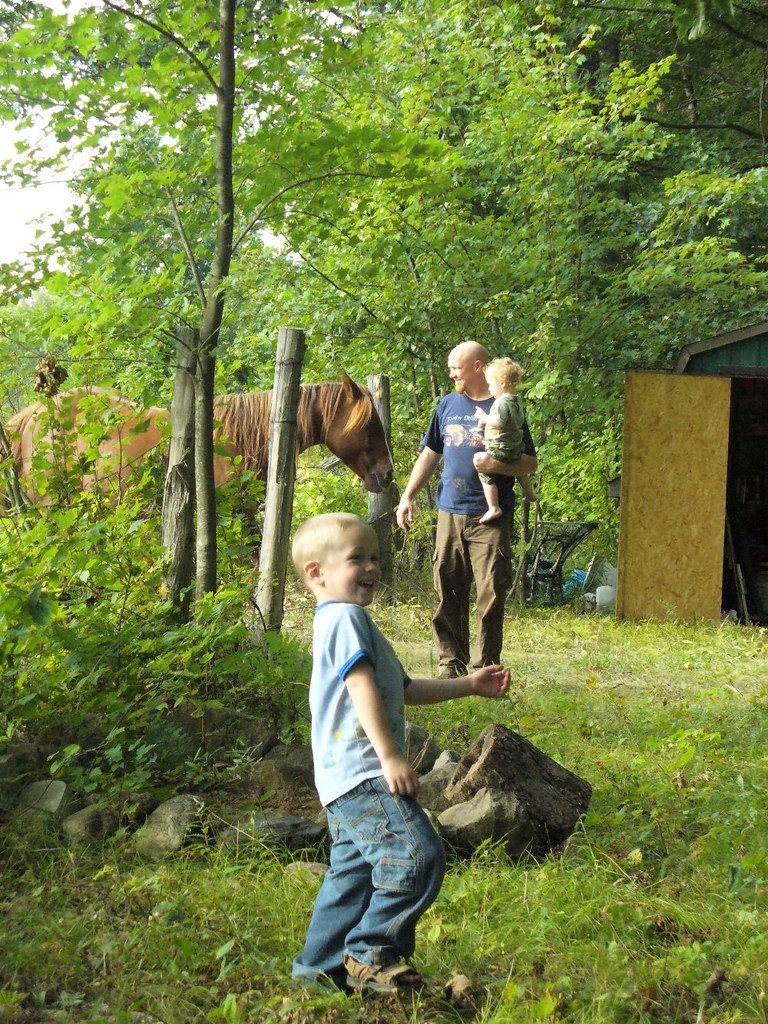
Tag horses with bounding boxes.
[2,370,393,566]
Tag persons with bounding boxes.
[292,512,511,993]
[478,356,537,524]
[397,341,537,679]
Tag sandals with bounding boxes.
[343,954,425,994]
